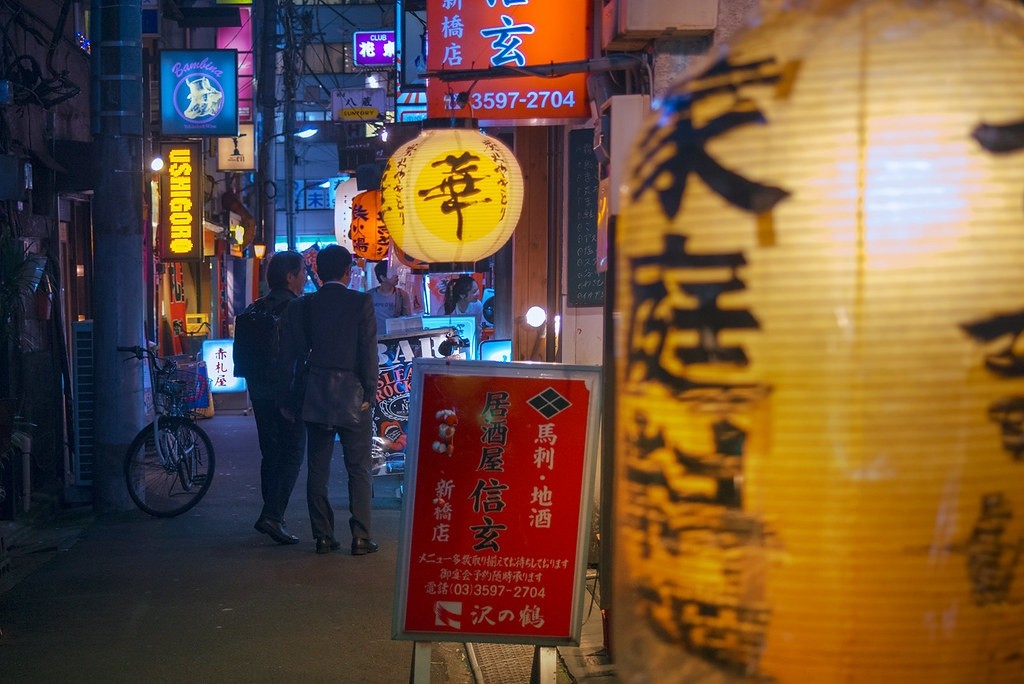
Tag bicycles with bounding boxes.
[172,319,211,355]
[114,344,217,518]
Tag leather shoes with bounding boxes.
[351,538,378,556]
[315,537,340,554]
[254,518,300,545]
[281,519,287,526]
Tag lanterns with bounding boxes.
[607,1,1023,684]
[333,126,524,264]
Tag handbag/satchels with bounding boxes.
[302,366,366,429]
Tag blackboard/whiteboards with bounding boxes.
[568,128,605,308]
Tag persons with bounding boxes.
[281,244,379,556]
[435,275,484,342]
[363,261,412,335]
[231,250,309,546]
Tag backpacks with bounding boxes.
[243,297,286,358]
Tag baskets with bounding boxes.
[154,366,212,415]
[179,331,208,357]
